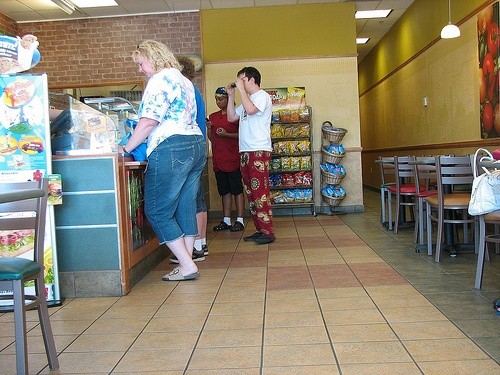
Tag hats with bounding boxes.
[214,86,229,97]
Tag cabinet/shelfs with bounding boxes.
[267,85,317,217]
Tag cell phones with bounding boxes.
[205,118,209,122]
[231,83,236,88]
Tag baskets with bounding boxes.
[320,163,347,185]
[320,143,347,165]
[320,185,347,207]
[321,120,349,144]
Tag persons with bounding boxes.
[143,57,209,262]
[225,65,276,245]
[202,87,246,232]
[117,39,206,280]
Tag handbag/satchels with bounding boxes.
[467,148,500,216]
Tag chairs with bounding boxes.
[413,153,459,245]
[426,155,481,262]
[377,156,415,223]
[0,178,59,375]
[471,155,500,289]
[387,155,426,234]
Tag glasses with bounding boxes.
[136,44,144,49]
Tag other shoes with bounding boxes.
[243,232,263,242]
[169,247,206,264]
[202,245,209,256]
[255,234,276,244]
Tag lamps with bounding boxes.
[441,0,460,38]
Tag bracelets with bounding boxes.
[122,147,130,154]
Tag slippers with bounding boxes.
[229,221,245,232]
[212,220,232,231]
[162,266,200,281]
